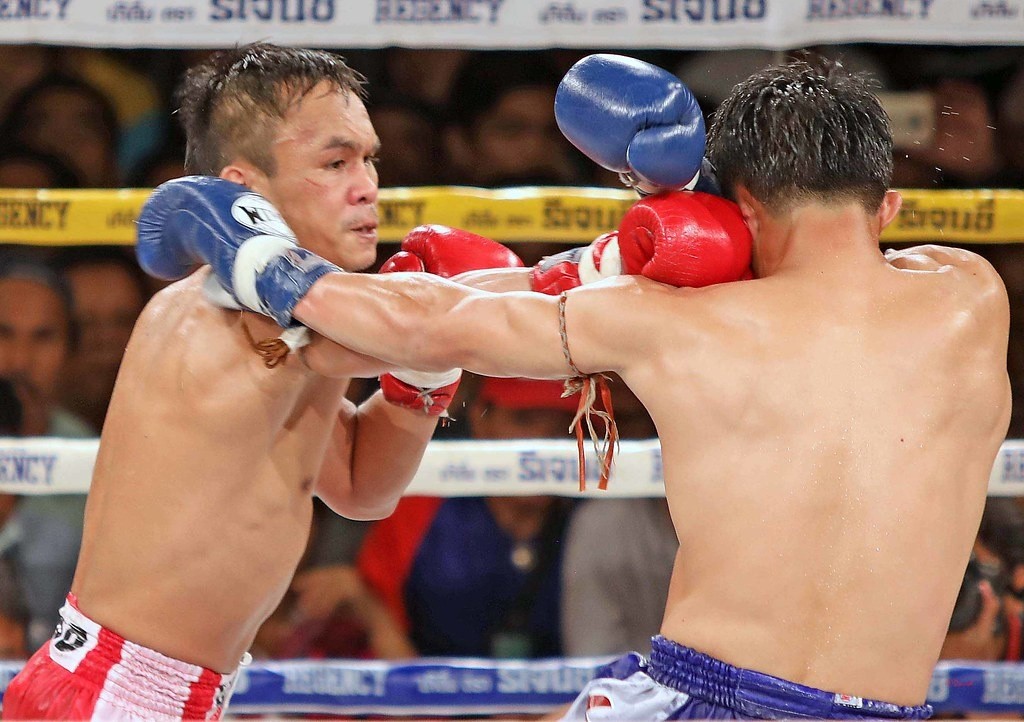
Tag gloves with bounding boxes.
[136,175,347,329]
[374,224,526,416]
[553,54,707,194]
[527,190,752,297]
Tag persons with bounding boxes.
[0,43,1024,661]
[135,55,1014,719]
[0,42,752,722]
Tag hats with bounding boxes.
[482,378,582,413]
